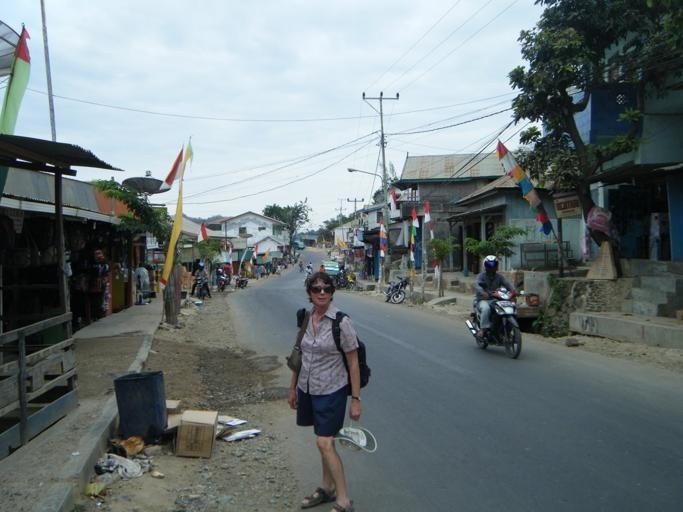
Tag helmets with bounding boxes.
[482,255,499,273]
[198,262,204,269]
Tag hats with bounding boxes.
[332,425,377,454]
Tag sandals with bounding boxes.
[333,500,354,512]
[301,487,336,509]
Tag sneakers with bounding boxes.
[477,330,484,337]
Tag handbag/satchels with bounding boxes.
[286,349,301,373]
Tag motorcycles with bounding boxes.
[235,275,248,290]
[465,287,529,359]
[194,275,208,299]
[382,272,411,304]
[216,274,230,292]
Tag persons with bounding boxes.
[471,254,518,338]
[282,270,363,511]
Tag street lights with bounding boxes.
[347,167,390,270]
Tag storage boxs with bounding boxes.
[175,410,218,458]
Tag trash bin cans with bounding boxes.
[113,370,168,445]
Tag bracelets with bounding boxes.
[350,395,361,401]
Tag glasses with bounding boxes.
[311,286,334,294]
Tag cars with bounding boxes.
[321,261,339,277]
[292,241,305,249]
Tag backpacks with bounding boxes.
[296,309,371,387]
